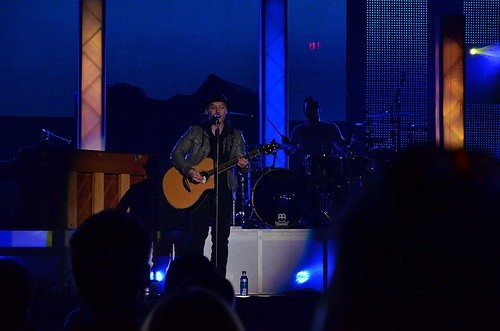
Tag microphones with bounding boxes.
[213,114,221,119]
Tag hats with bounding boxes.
[207,91,230,107]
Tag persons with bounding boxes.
[169,92,250,280]
[285,95,360,181]
[1,143,500,331]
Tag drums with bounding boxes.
[330,152,365,188]
[293,156,329,187]
[251,168,322,229]
[240,166,276,206]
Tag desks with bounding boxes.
[202,226,339,294]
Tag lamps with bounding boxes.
[39,128,72,146]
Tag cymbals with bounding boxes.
[246,143,302,150]
[367,111,415,116]
[366,136,388,143]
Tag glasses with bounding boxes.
[305,107,318,114]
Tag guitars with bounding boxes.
[162,142,280,211]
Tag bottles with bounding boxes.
[240,271,248,295]
